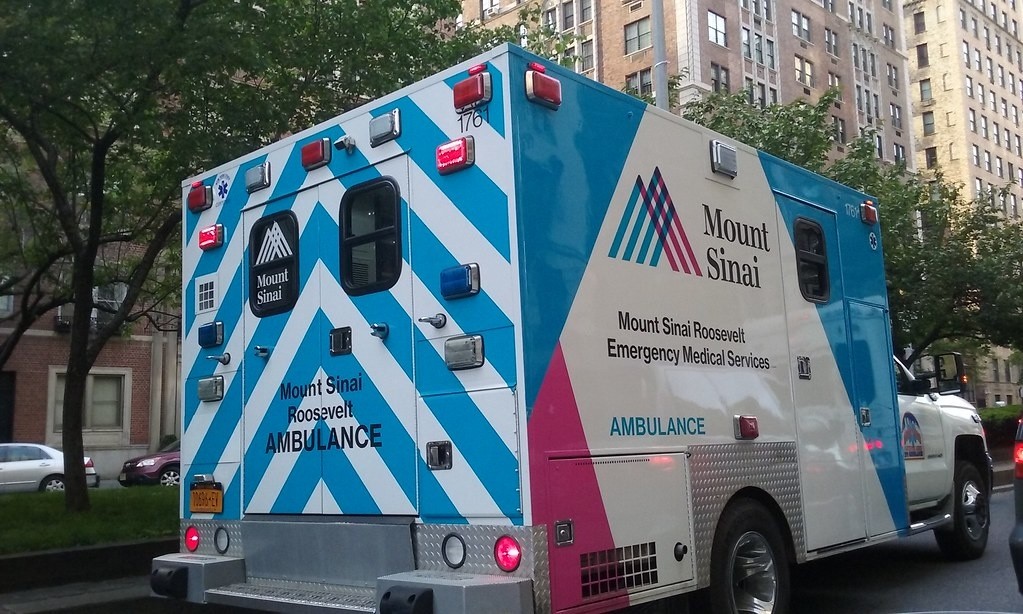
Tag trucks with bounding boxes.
[149,41,995,614]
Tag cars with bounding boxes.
[116,438,181,488]
[0,443,101,496]
